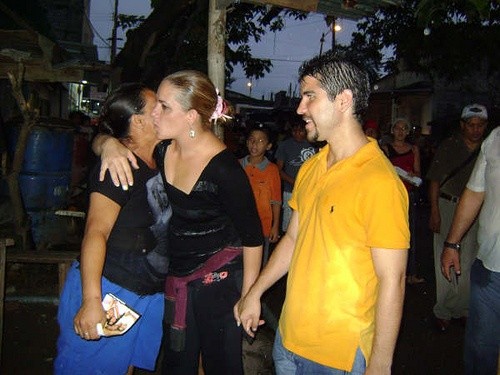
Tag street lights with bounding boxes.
[247,77,252,96]
[319,24,342,57]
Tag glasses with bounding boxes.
[249,138,266,144]
[394,126,408,131]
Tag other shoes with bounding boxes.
[451,316,467,333]
[436,318,450,333]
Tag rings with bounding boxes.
[82,332,89,336]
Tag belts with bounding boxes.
[439,192,457,202]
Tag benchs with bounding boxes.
[4,248,81,304]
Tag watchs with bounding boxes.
[444,242,462,251]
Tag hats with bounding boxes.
[391,118,411,134]
[461,104,488,121]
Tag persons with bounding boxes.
[54,50,500,374]
